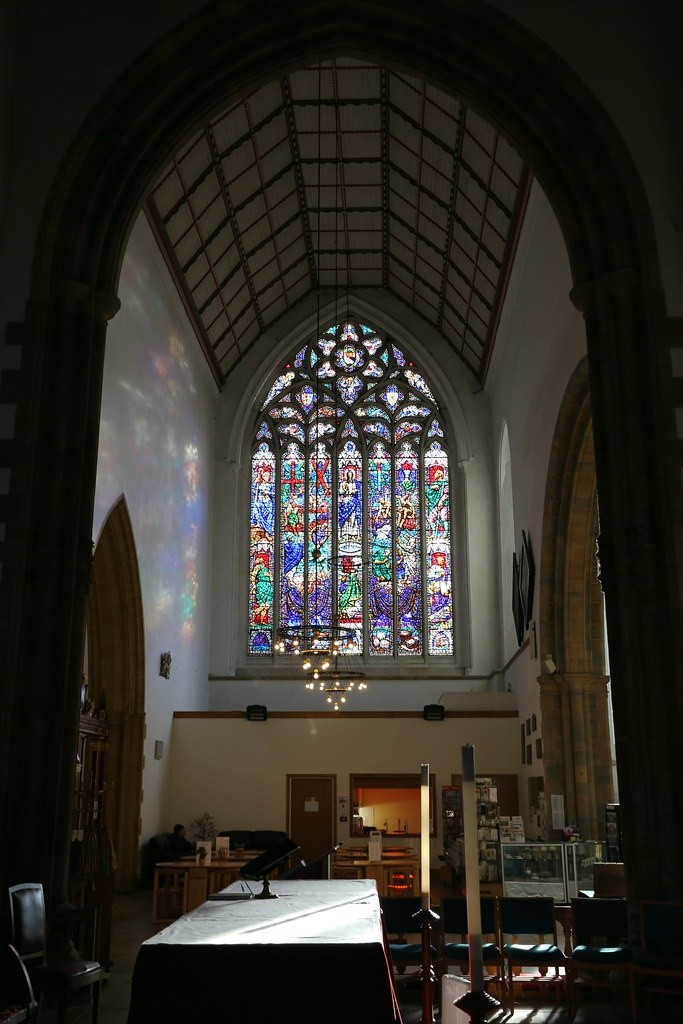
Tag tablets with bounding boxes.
[238,839,301,878]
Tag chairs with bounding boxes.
[8,884,103,1024]
[378,895,441,1000]
[569,863,683,1019]
[433,895,508,1014]
[499,895,570,1014]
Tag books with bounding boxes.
[442,787,525,897]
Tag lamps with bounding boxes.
[246,704,267,721]
[421,762,430,909]
[542,658,556,676]
[305,128,368,710]
[460,741,486,991]
[423,704,445,721]
[240,838,301,898]
[276,56,356,680]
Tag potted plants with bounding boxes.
[198,846,206,864]
[235,842,245,853]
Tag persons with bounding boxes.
[167,824,192,852]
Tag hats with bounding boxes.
[173,824,183,833]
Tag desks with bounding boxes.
[153,848,269,923]
[127,879,406,1024]
[335,846,419,897]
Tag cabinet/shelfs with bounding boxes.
[501,844,564,884]
[566,842,607,883]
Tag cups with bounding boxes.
[572,834,579,843]
[570,837,576,844]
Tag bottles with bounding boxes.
[522,847,558,878]
[384,818,408,832]
[486,812,496,826]
